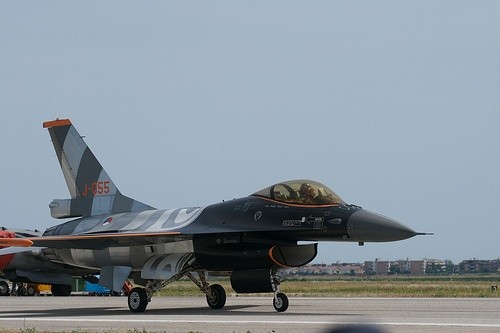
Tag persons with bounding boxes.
[299,182,316,199]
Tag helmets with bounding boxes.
[300,183,315,197]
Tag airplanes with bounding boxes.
[0,226,93,297]
[0,118,434,313]
